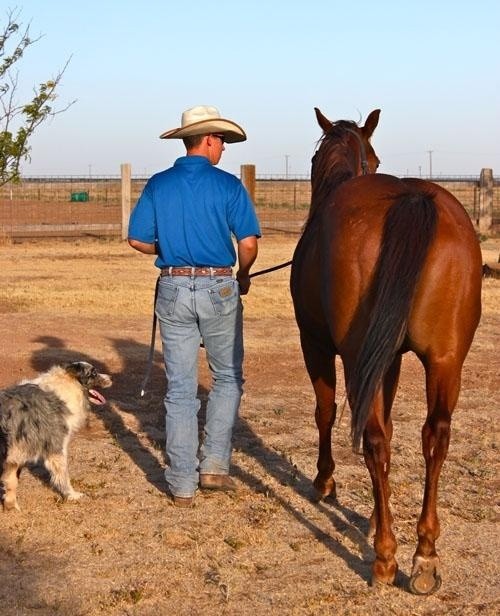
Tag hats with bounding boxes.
[160,104,247,144]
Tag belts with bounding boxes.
[160,267,234,277]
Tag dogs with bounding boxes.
[0,361,115,510]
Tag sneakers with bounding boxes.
[174,495,195,508]
[199,473,238,492]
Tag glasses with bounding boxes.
[214,133,226,145]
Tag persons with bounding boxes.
[124,103,264,509]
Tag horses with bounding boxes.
[291,108,483,594]
